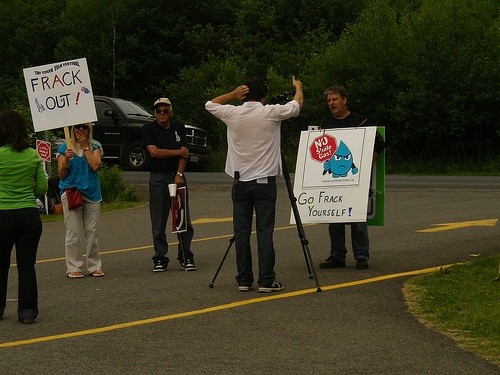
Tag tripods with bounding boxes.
[209,148,322,292]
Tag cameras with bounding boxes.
[270,90,296,105]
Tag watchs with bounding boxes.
[177,172,183,177]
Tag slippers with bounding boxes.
[92,270,105,276]
[67,272,84,278]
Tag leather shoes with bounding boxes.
[356,259,369,269]
[319,255,346,269]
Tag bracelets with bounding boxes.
[84,147,90,152]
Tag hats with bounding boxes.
[154,97,172,106]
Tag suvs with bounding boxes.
[56,95,209,170]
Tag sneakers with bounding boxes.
[153,259,168,272]
[258,280,284,292]
[66,186,84,211]
[238,281,252,291]
[180,258,197,270]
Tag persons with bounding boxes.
[204,75,305,293]
[0,109,51,325]
[56,122,105,278]
[317,86,386,270]
[142,97,198,272]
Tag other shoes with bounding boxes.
[18,311,34,323]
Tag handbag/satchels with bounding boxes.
[170,185,188,234]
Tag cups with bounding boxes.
[169,183,178,198]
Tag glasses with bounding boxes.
[74,124,89,129]
[155,109,169,113]
